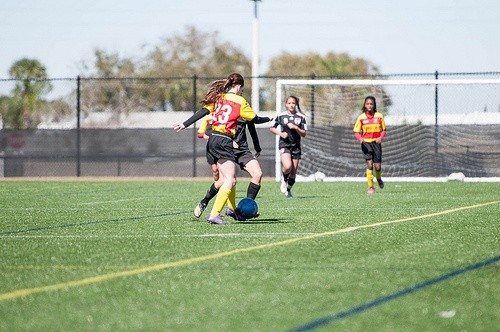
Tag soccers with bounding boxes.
[237,198,258,219]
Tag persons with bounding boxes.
[193,118,263,219]
[352,96,386,194]
[269,96,307,198]
[172,73,274,224]
[198,114,219,182]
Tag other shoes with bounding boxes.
[377,178,384,188]
[280,179,288,194]
[368,187,375,194]
[285,191,294,198]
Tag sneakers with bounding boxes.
[207,214,230,225]
[225,208,245,221]
[193,201,205,218]
